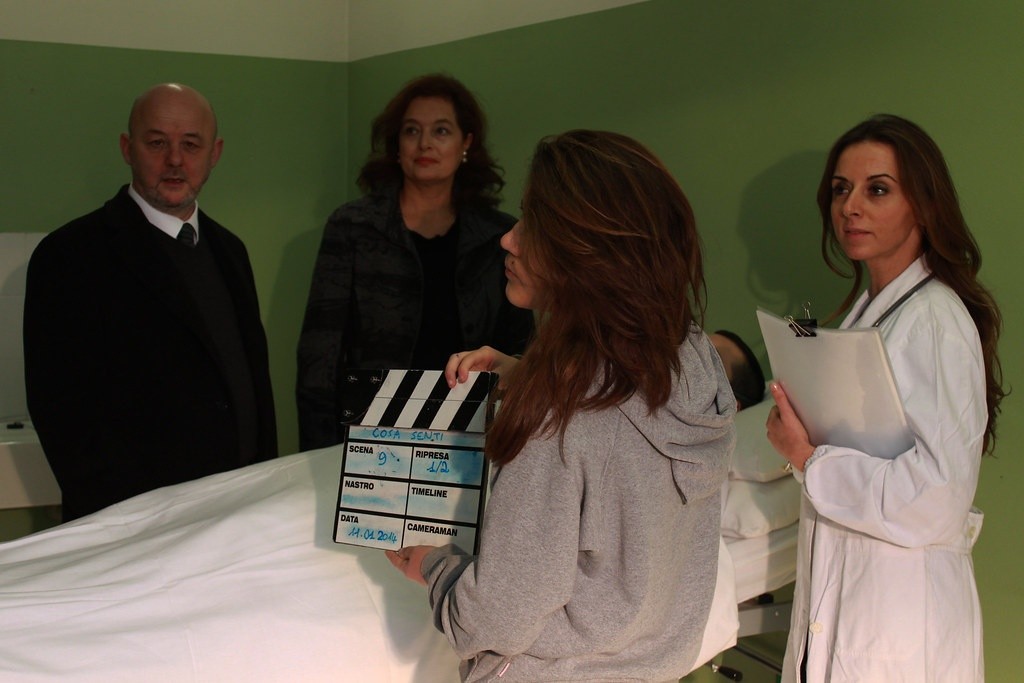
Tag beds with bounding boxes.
[0,378,803,683]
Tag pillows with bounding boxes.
[709,383,802,540]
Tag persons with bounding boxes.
[765,114,1005,683]
[295,74,535,453]
[385,130,738,683]
[22,83,280,523]
[708,330,765,415]
